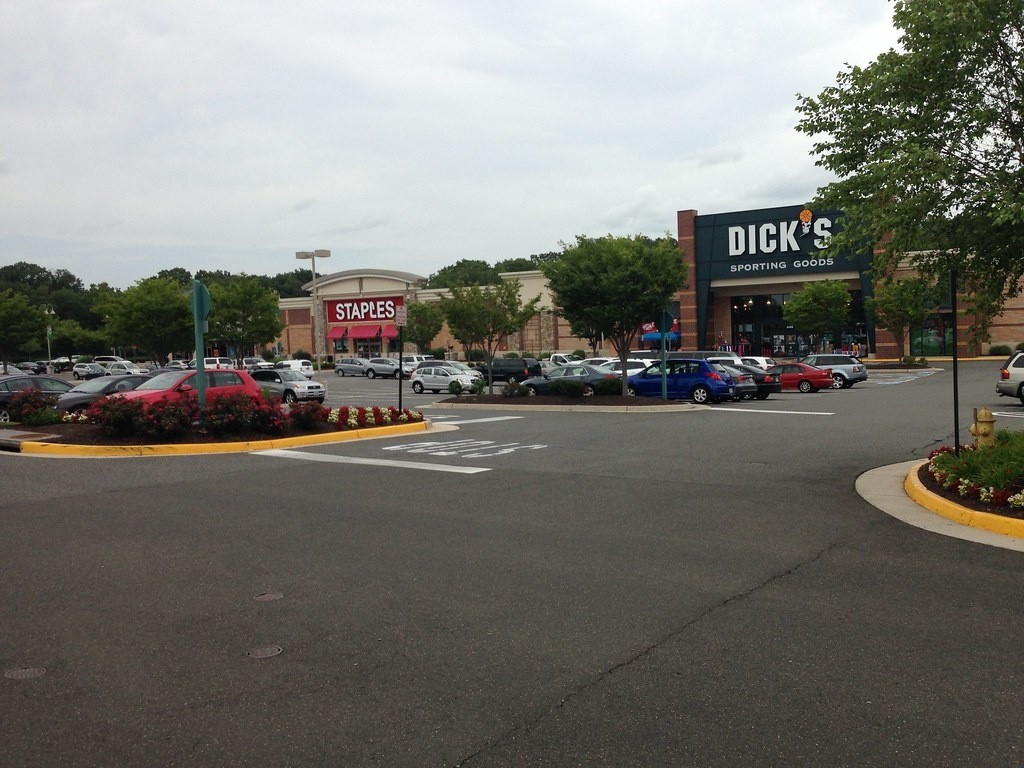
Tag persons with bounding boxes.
[842,342,848,354]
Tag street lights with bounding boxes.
[44,308,56,366]
[296,250,332,382]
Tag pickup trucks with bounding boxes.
[542,353,585,366]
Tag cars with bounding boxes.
[157,361,189,369]
[578,348,777,370]
[672,364,757,402]
[52,357,66,364]
[0,364,28,376]
[519,365,621,398]
[409,366,475,394]
[149,369,174,375]
[914,329,953,349]
[91,369,264,423]
[66,355,80,363]
[763,363,834,393]
[253,357,274,369]
[537,361,559,373]
[0,375,77,422]
[398,355,424,367]
[0,361,61,374]
[729,364,782,400]
[621,359,736,403]
[51,375,170,413]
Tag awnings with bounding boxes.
[641,332,680,341]
[325,327,346,339]
[346,326,381,338]
[381,325,400,338]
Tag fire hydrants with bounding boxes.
[969,405,997,450]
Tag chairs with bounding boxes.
[679,367,684,373]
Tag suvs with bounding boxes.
[233,358,259,370]
[798,354,869,389]
[413,360,484,384]
[995,351,1024,405]
[364,358,415,379]
[189,357,236,370]
[334,358,370,377]
[105,362,150,375]
[92,356,132,365]
[72,363,107,379]
[236,368,325,404]
[472,358,542,384]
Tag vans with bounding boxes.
[275,360,315,380]
